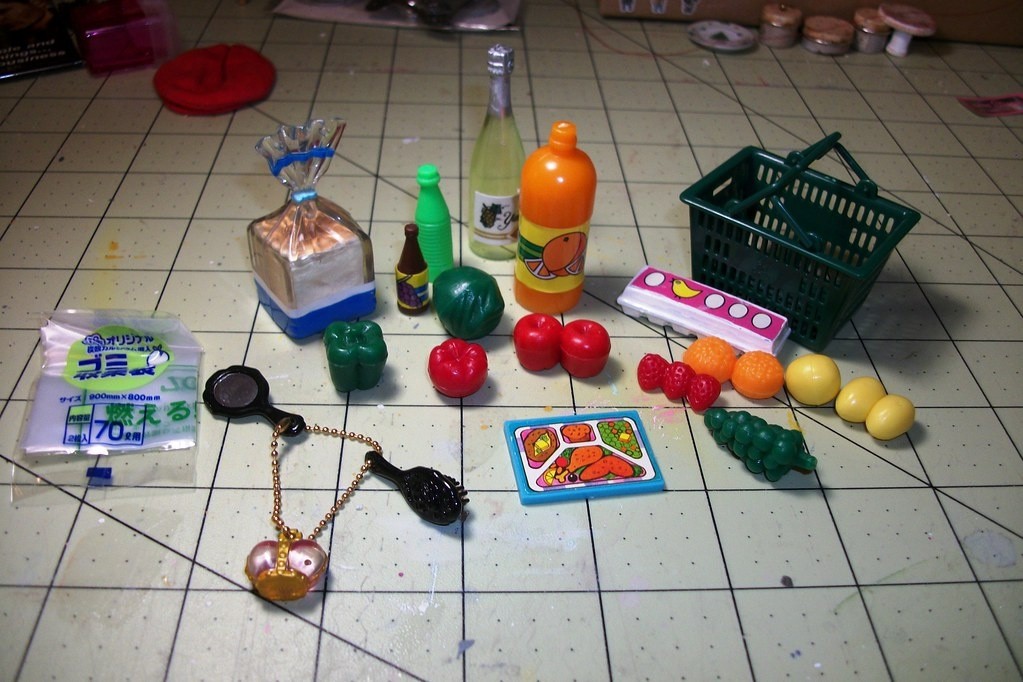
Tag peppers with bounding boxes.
[323,320,388,392]
[427,338,487,398]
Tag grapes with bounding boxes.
[704,408,817,482]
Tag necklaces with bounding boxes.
[244,420,381,599]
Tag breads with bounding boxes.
[248,204,366,315]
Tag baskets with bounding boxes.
[679,131,922,355]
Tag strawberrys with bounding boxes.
[638,354,721,412]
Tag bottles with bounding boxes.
[469,40,526,259]
[516,121,596,314]
[396,223,430,315]
[416,165,455,283]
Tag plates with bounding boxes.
[688,20,754,50]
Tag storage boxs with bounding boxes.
[599,0,1023,48]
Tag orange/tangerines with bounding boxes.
[682,336,786,399]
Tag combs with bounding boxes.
[365,452,469,526]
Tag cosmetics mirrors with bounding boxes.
[203,365,306,437]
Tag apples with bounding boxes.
[513,313,610,378]
